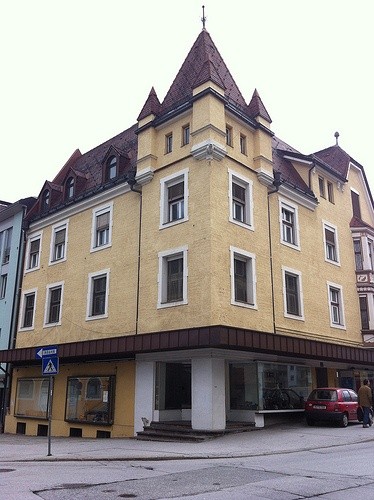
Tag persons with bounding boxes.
[358,378,374,428]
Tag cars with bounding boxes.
[305,387,374,430]
[268,388,307,410]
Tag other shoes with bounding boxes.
[363,424,369,428]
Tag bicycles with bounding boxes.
[261,380,290,411]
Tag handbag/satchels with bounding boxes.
[357,407,365,421]
